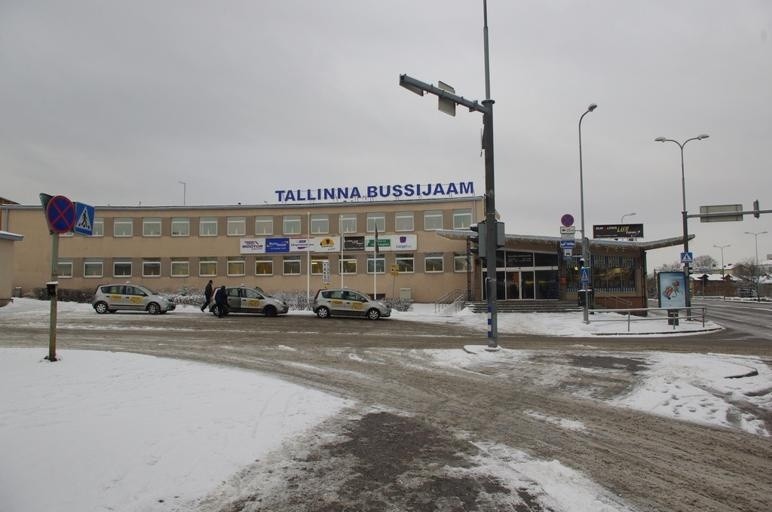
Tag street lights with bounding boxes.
[743,229,769,303]
[711,243,731,301]
[578,103,598,324]
[619,212,637,239]
[655,131,709,324]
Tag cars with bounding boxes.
[207,287,291,317]
[92,283,178,315]
[313,288,395,320]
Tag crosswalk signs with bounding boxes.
[680,252,693,264]
[72,202,95,235]
[581,267,590,283]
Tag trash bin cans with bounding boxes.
[668,309,679,329]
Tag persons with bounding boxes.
[201,279,213,313]
[215,286,228,318]
